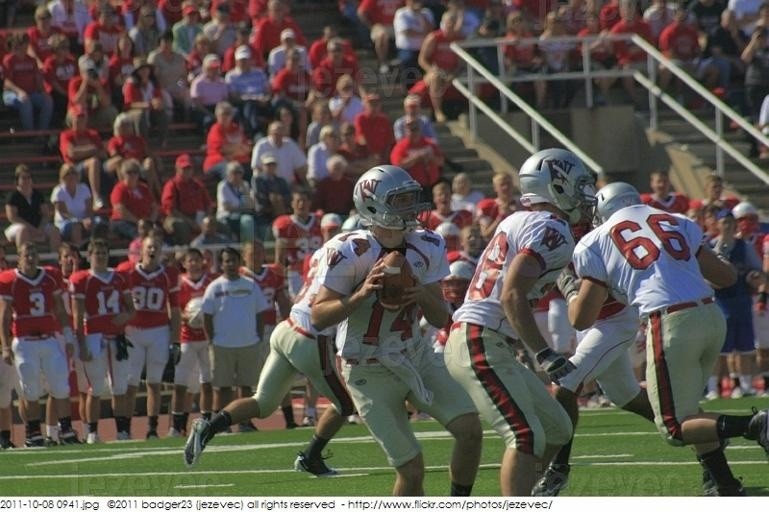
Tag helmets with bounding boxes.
[351,164,434,234]
[434,222,461,238]
[441,261,476,307]
[592,181,642,230]
[518,147,598,227]
[731,201,759,222]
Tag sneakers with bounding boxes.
[743,407,768,458]
[182,417,211,467]
[293,451,340,478]
[0,417,330,448]
[695,447,744,497]
[531,463,571,496]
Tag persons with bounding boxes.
[528,222,728,496]
[2,0,769,451]
[311,165,482,497]
[444,147,598,497]
[185,213,373,474]
[556,181,769,497]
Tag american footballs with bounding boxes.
[374,250,416,313]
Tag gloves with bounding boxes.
[114,333,134,361]
[555,264,580,306]
[535,346,580,389]
[162,342,181,383]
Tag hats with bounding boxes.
[716,209,734,220]
[177,94,382,171]
[191,1,346,89]
[320,213,342,228]
